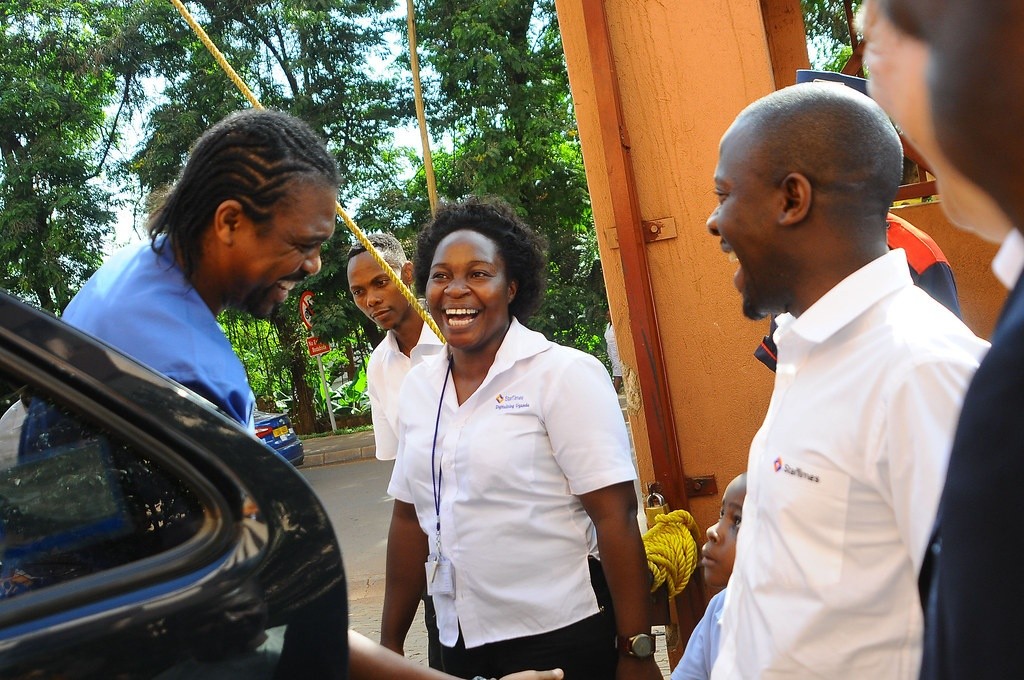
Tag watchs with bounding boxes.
[614,633,656,659]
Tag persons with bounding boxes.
[751,70,962,375]
[704,87,990,679]
[603,311,623,393]
[379,193,667,680]
[17,110,566,680]
[851,0,1024,678]
[346,233,447,460]
[669,474,748,680]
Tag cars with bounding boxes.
[252,409,305,467]
